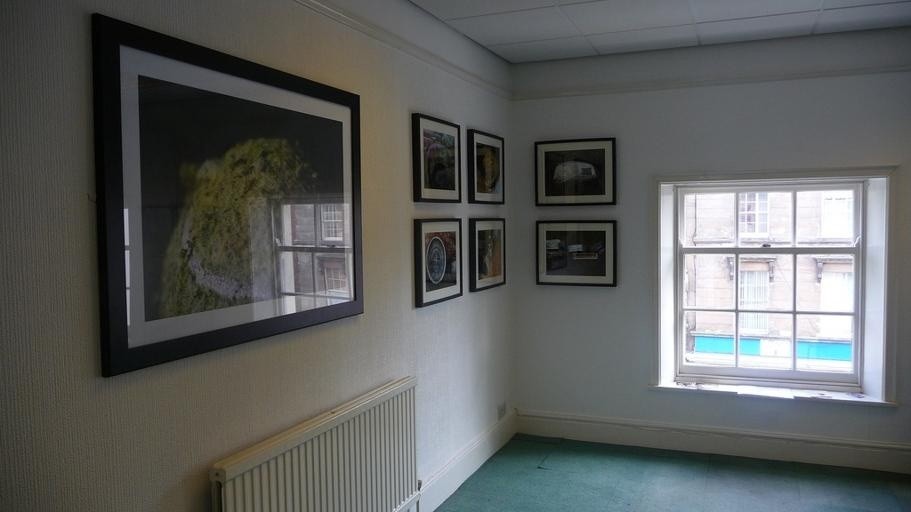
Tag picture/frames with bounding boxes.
[536,218,618,287]
[534,137,618,207]
[469,218,507,293]
[413,217,464,309]
[87,11,365,377]
[467,129,507,205]
[413,112,464,203]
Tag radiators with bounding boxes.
[208,372,423,510]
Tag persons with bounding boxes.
[425,133,455,189]
[429,246,444,281]
